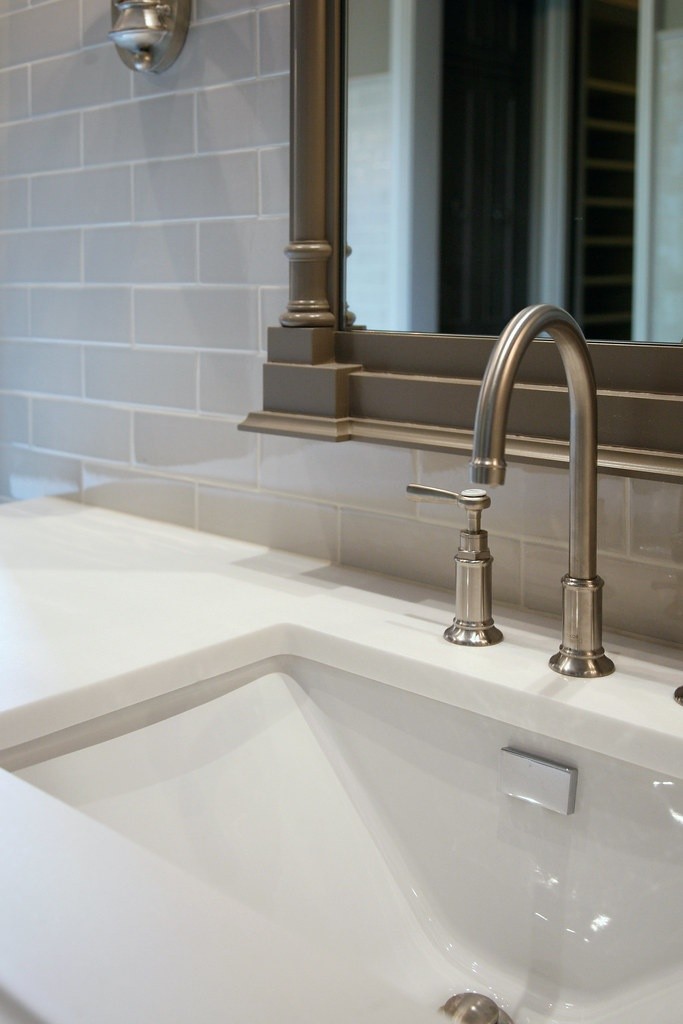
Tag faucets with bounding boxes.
[471,301,617,678]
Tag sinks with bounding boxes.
[1,625,682,1024]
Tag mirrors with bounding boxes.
[338,0,683,344]
